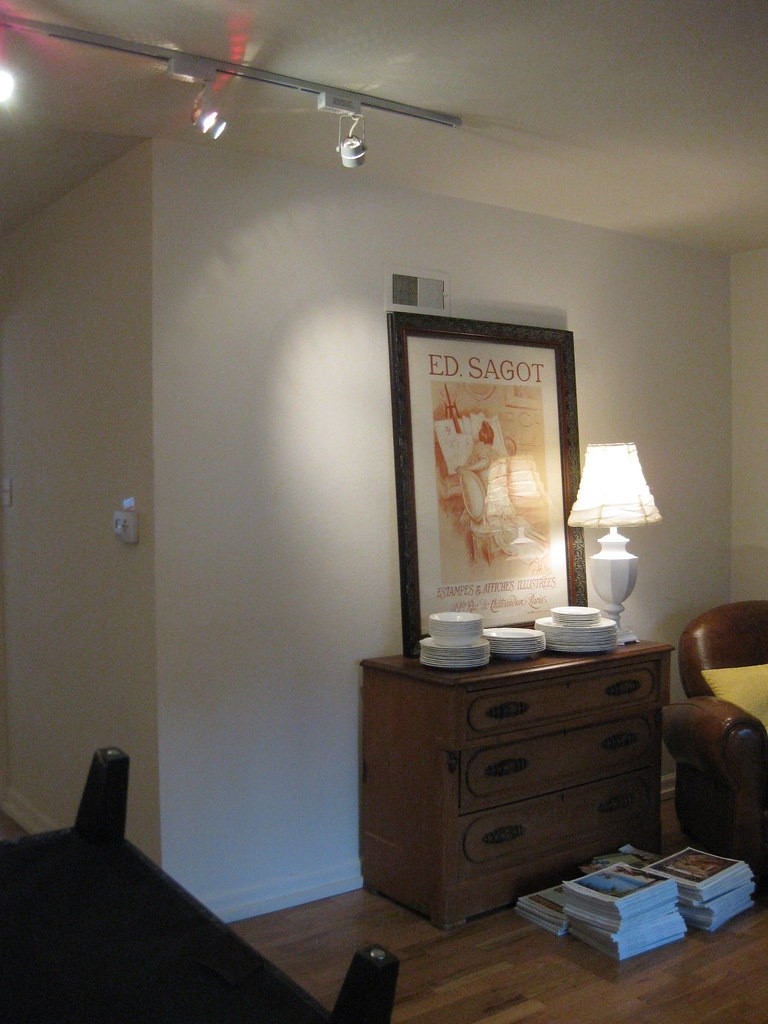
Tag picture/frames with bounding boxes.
[386,311,588,660]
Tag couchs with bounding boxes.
[660,601,768,900]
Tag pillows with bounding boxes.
[700,664,768,736]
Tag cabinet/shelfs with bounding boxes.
[359,639,677,930]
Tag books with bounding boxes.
[514,844,688,961]
[642,847,756,930]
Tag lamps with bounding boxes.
[167,59,228,140]
[318,93,368,169]
[567,442,662,648]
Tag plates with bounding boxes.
[420,606,618,669]
